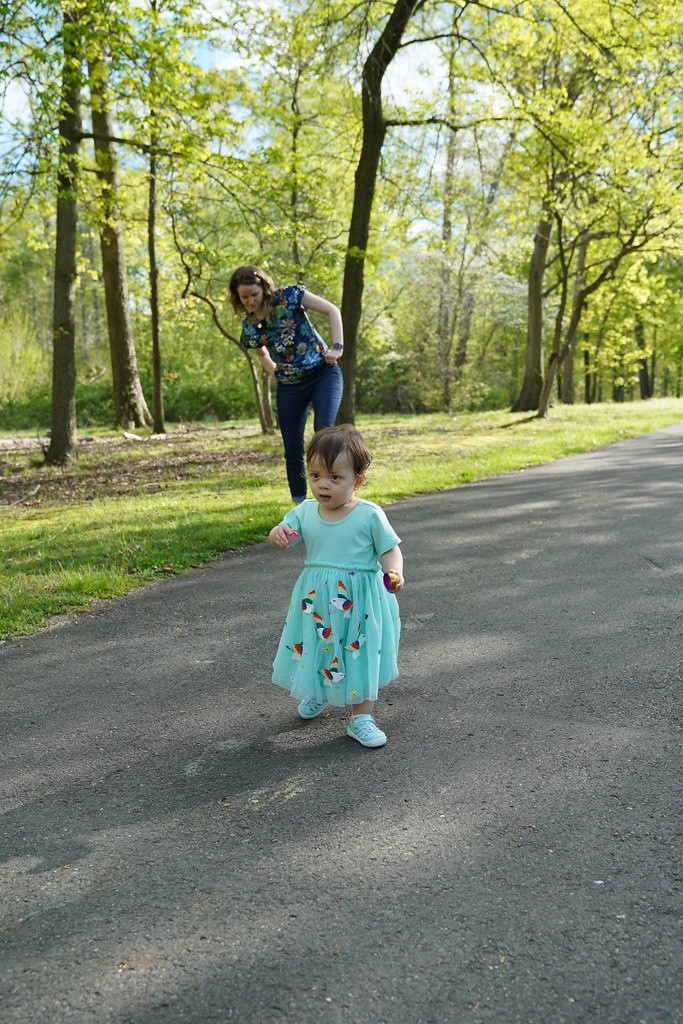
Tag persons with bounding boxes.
[227,265,344,507]
[268,423,403,748]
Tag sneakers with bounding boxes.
[347,715,387,749]
[298,697,326,719]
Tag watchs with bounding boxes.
[333,343,343,350]
